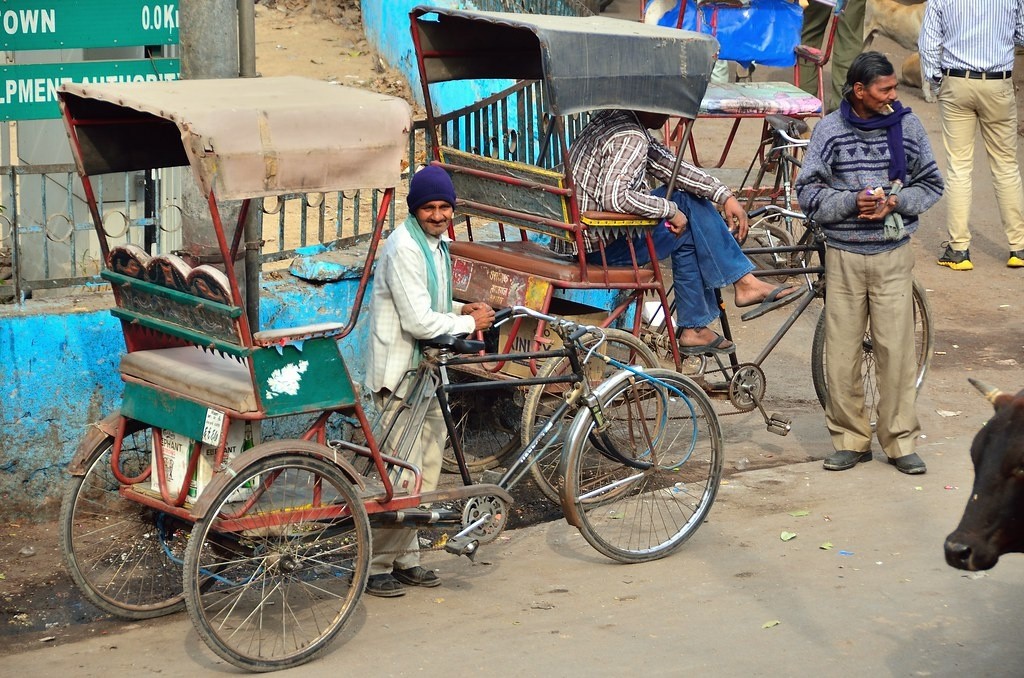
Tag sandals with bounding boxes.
[347,566,441,597]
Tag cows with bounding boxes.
[942,376,1024,573]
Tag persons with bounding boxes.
[345,167,495,596]
[917,0,1024,270]
[551,110,808,355]
[798,0,866,113]
[795,48,945,474]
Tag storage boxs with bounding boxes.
[496,295,610,384]
[150,419,260,505]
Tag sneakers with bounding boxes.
[1006,250,1024,268]
[936,240,974,271]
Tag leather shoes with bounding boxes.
[888,453,927,474]
[823,450,872,470]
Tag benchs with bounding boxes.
[107,244,394,517]
[641,0,844,196]
[429,144,682,385]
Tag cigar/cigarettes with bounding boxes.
[886,103,894,113]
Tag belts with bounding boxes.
[942,68,1013,81]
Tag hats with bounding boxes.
[406,167,457,218]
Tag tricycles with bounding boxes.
[406,4,938,515]
[654,0,841,293]
[55,78,724,665]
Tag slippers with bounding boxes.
[741,283,810,321]
[679,331,736,356]
